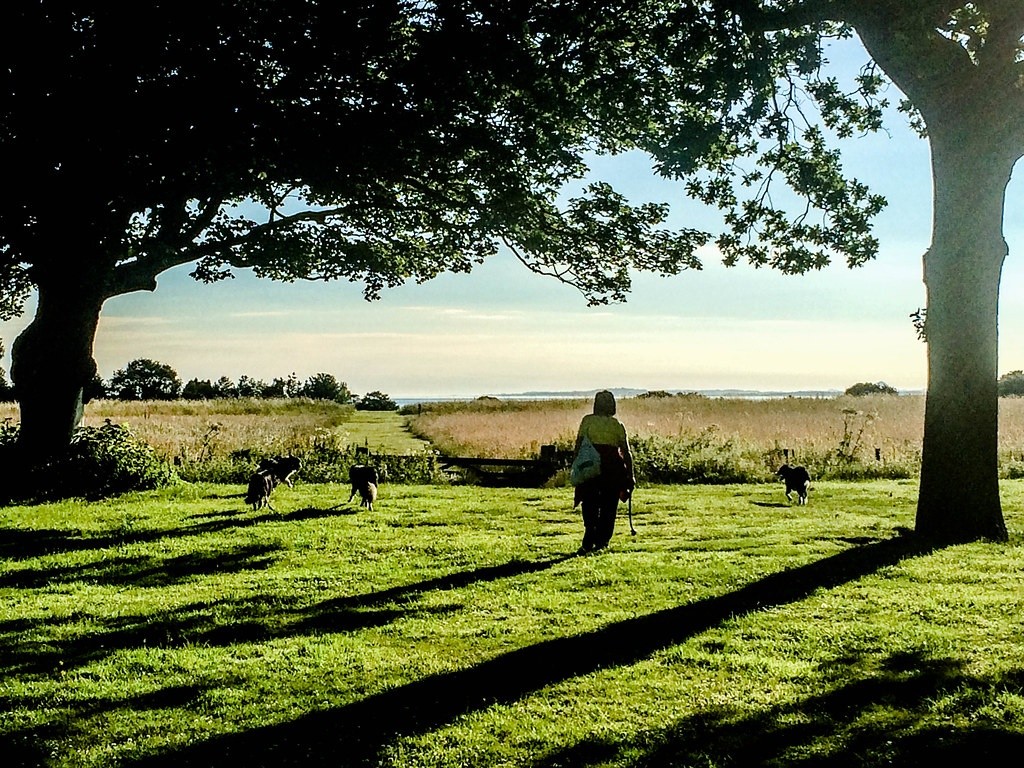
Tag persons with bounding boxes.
[572,390,636,555]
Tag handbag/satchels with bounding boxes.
[570,438,602,486]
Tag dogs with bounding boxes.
[776,465,811,506]
[347,463,379,512]
[244,455,302,511]
